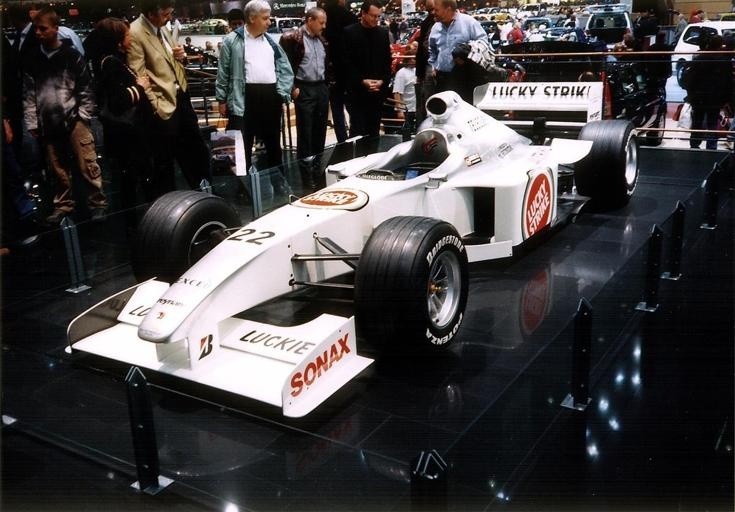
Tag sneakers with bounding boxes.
[300,154,324,190]
[272,180,294,195]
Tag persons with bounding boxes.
[602,2,733,152]
[215,0,398,176]
[394,1,587,152]
[3,2,213,256]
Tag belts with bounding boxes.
[295,77,327,87]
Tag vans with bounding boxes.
[268,16,303,33]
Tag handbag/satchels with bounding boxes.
[95,53,139,140]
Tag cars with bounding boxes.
[58,19,66,27]
[177,17,227,35]
[65,20,94,35]
